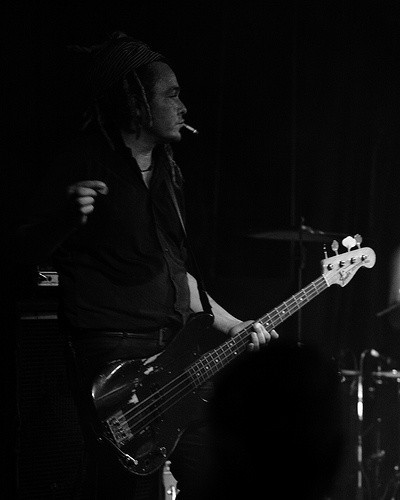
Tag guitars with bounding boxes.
[86,233,377,477]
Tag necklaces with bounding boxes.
[139,163,153,173]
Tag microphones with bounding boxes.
[182,124,200,136]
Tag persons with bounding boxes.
[43,37,281,500]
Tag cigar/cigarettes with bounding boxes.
[182,123,200,135]
